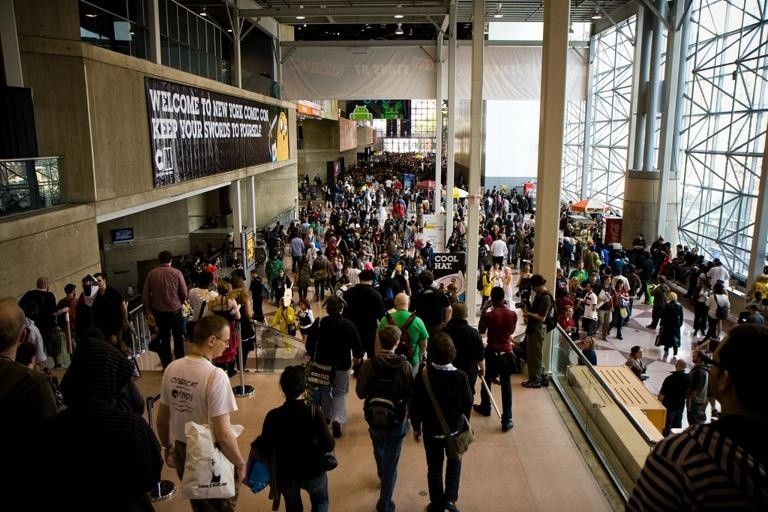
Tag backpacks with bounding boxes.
[255,277,271,300]
[363,374,409,438]
[22,290,46,322]
[477,273,491,291]
[385,310,414,361]
[298,269,312,289]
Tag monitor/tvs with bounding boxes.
[110,227,134,244]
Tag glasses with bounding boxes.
[697,348,724,371]
[215,335,230,349]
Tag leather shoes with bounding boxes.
[473,404,490,416]
[427,503,432,512]
[445,501,460,512]
[521,380,541,388]
[502,419,513,432]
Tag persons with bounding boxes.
[0,149,768,512]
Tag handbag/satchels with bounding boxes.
[288,324,296,337]
[655,335,661,346]
[716,303,728,320]
[306,361,335,389]
[547,313,557,333]
[314,446,337,472]
[446,412,475,463]
[242,437,270,495]
[313,269,328,280]
[619,295,631,308]
[620,307,628,319]
[181,421,245,501]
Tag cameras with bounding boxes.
[514,297,529,308]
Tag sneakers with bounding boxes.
[541,374,549,385]
[332,420,342,438]
[646,324,654,328]
[602,336,609,342]
[616,335,622,340]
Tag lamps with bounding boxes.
[393,2,403,19]
[199,4,207,17]
[494,3,503,18]
[409,27,414,36]
[591,4,602,20]
[569,22,574,33]
[228,23,232,32]
[129,23,135,35]
[295,5,306,20]
[394,19,403,35]
